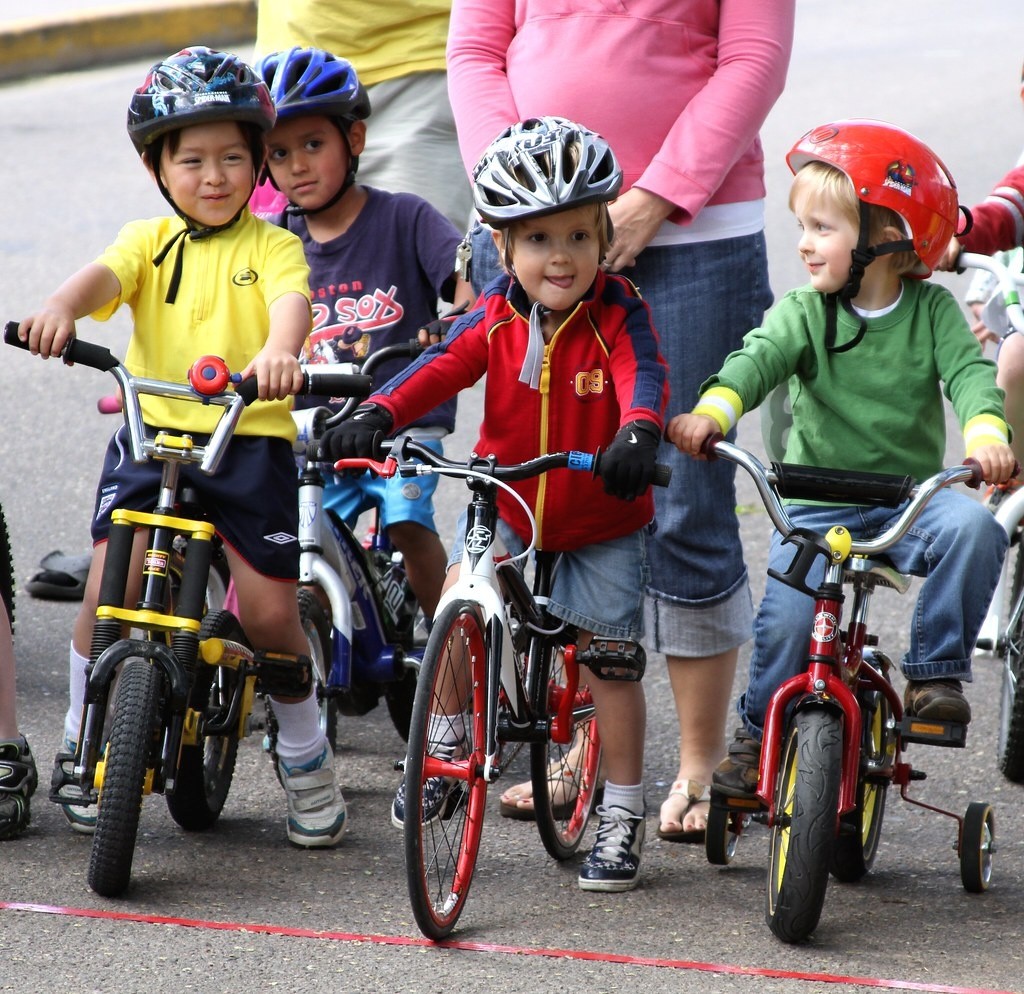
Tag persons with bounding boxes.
[18,47,346,847]
[319,116,670,890]
[932,150,1024,520]
[247,45,475,631]
[443,0,793,838]
[256,0,476,239]
[0,595,38,842]
[667,120,1013,798]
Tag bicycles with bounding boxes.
[3,319,373,895]
[288,333,455,755]
[305,428,674,941]
[941,244,1024,785]
[662,421,1017,943]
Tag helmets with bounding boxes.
[472,117,623,230]
[127,45,277,145]
[253,47,373,130]
[786,118,960,280]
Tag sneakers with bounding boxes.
[391,732,469,829]
[0,733,37,838]
[904,676,972,728]
[58,729,100,834]
[577,796,650,891]
[981,476,1024,527]
[710,730,766,801]
[262,726,347,848]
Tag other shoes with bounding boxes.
[25,546,92,602]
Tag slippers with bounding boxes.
[656,778,713,841]
[499,758,587,821]
